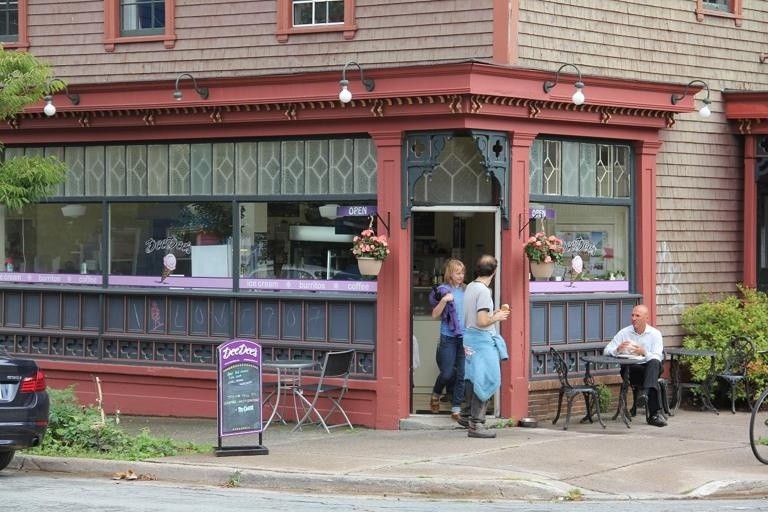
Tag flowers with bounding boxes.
[523,231,565,263]
[350,229,391,259]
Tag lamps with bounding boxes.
[173,73,209,102]
[338,61,375,104]
[543,63,585,106]
[43,79,80,117]
[671,79,712,119]
[60,204,87,219]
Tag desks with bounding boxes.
[663,348,720,416]
[262,359,317,432]
[579,354,643,428]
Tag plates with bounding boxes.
[616,355,635,358]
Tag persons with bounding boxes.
[464,254,511,437]
[429,259,468,421]
[603,305,667,426]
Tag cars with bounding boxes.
[246,264,358,279]
[1,352,50,474]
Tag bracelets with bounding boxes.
[442,299,447,303]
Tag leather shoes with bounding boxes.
[458,417,470,427]
[648,415,668,426]
[468,423,496,438]
[636,391,648,406]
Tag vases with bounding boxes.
[356,257,382,276]
[530,262,554,279]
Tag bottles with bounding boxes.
[80,258,87,274]
[437,268,443,286]
[432,268,437,285]
[6,257,14,272]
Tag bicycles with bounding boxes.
[749,386,768,466]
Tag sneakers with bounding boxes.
[430,396,439,413]
[452,413,461,419]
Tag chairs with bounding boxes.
[623,353,674,423]
[550,346,607,429]
[290,349,357,434]
[714,336,755,414]
[261,380,288,432]
